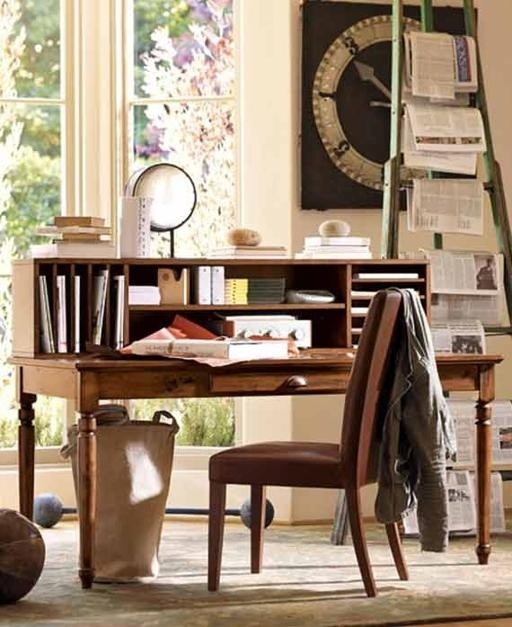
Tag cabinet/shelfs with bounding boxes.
[14,259,431,359]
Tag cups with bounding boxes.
[119,195,150,258]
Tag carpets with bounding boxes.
[0,520,512,627]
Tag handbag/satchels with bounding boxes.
[59,405,180,584]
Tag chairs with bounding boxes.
[206,291,430,599]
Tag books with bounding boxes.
[351,308,370,315]
[112,275,125,350]
[75,276,80,354]
[34,227,111,234]
[351,328,363,333]
[305,236,369,245]
[93,276,104,346]
[98,270,108,345]
[351,290,419,298]
[352,273,418,279]
[53,217,104,227]
[57,275,67,354]
[353,344,358,350]
[38,276,51,354]
[131,339,288,359]
[202,246,288,259]
[295,245,372,260]
[52,239,108,245]
[58,233,100,239]
[31,245,114,258]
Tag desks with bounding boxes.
[13,354,503,591]
